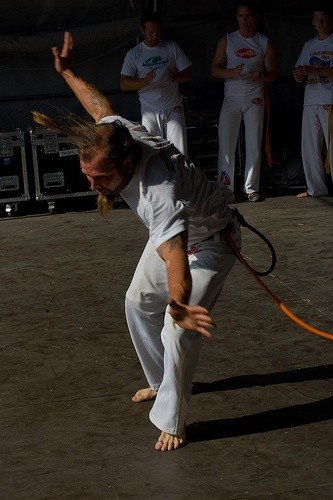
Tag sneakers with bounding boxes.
[242,192,265,203]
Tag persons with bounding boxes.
[208,0,276,205]
[288,3,333,201]
[119,12,200,166]
[52,22,249,454]
